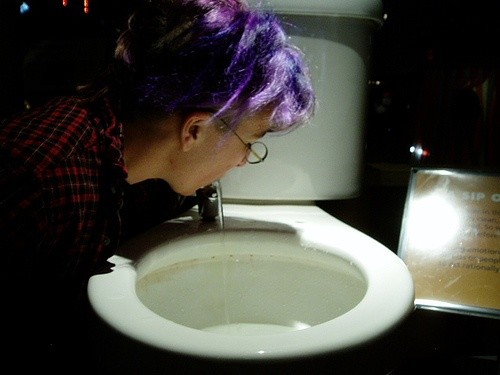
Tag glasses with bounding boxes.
[211,110,267,164]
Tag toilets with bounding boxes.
[85,0,416,363]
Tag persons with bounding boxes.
[2,0,317,285]
